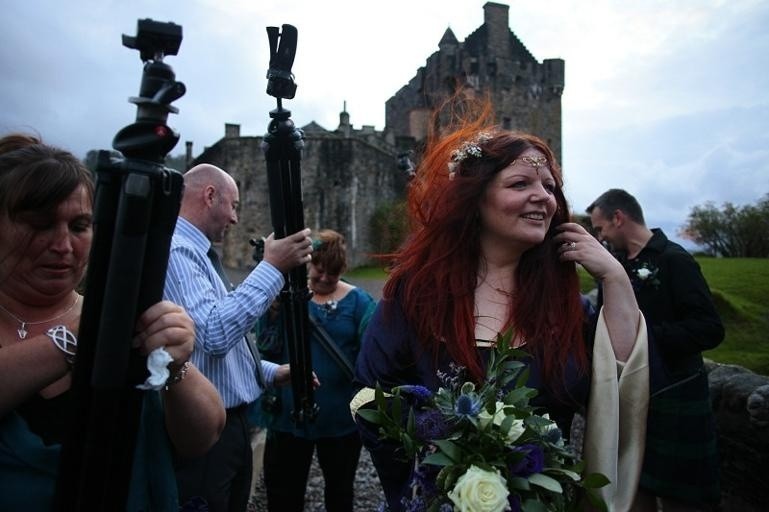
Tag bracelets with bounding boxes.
[43,324,77,367]
[165,361,194,392]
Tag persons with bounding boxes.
[162,163,321,512]
[255,230,377,512]
[356,77,650,512]
[586,189,724,511]
[1,135,226,512]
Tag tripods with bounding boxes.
[250,236,360,398]
[261,24,320,428]
[49,19,186,512]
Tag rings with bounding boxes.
[570,242,576,250]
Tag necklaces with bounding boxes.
[477,274,520,300]
[2,290,80,338]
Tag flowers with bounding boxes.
[356,324,610,512]
[637,261,660,286]
